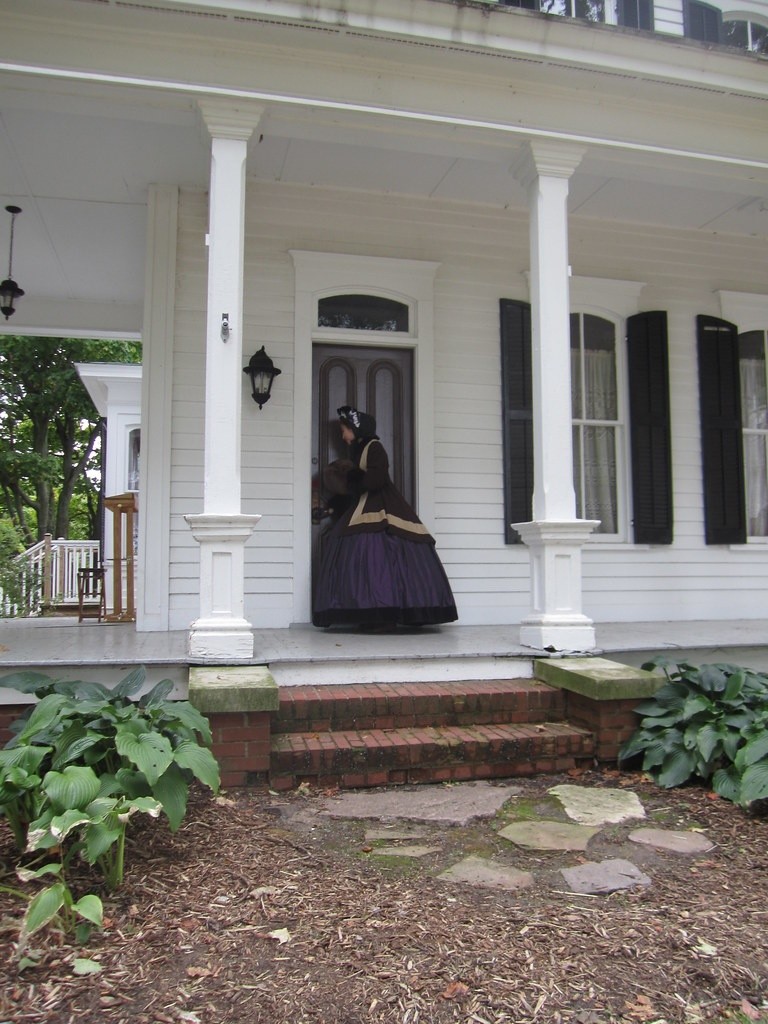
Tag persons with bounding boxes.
[312,406,460,632]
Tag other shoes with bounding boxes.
[358,616,401,634]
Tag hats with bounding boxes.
[337,406,380,439]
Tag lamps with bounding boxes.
[0,205,25,321]
[242,346,281,408]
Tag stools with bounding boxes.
[76,568,108,623]
